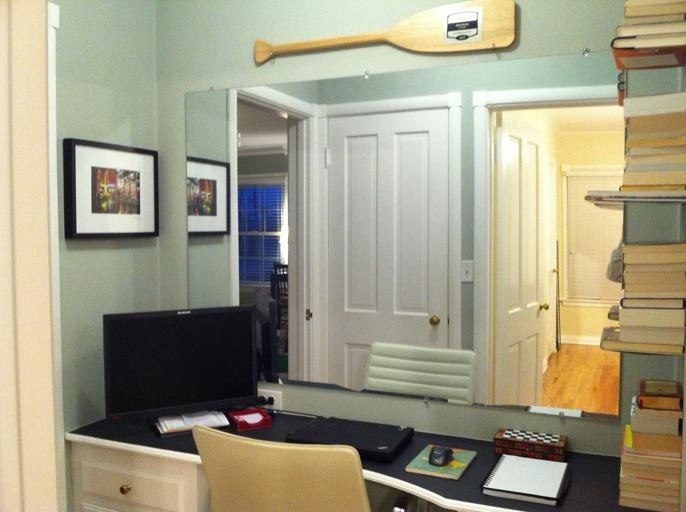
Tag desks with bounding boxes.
[62,409,642,512]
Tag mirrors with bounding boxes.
[185,52,626,424]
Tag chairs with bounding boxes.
[191,425,371,512]
[364,342,475,402]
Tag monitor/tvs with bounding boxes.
[102,304,259,420]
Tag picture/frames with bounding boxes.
[63,139,159,239]
[184,157,230,234]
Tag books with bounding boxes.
[480,453,571,506]
[584,0,686,356]
[405,443,478,481]
[618,379,684,512]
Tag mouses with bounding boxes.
[429,446,453,467]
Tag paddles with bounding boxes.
[254,0,516,64]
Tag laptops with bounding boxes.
[286,415,414,463]
